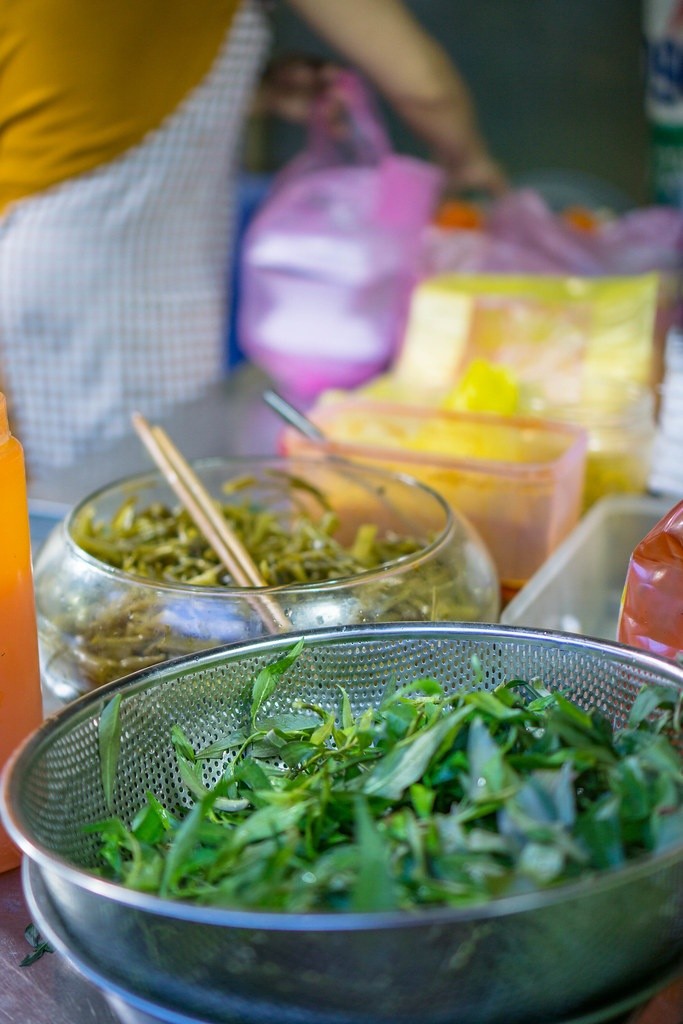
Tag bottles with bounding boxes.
[0,391,51,874]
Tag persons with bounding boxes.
[0,0,512,520]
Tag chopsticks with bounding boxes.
[130,410,320,687]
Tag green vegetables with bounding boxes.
[75,637,683,912]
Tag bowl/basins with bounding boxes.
[32,456,500,707]
[21,863,683,1024]
